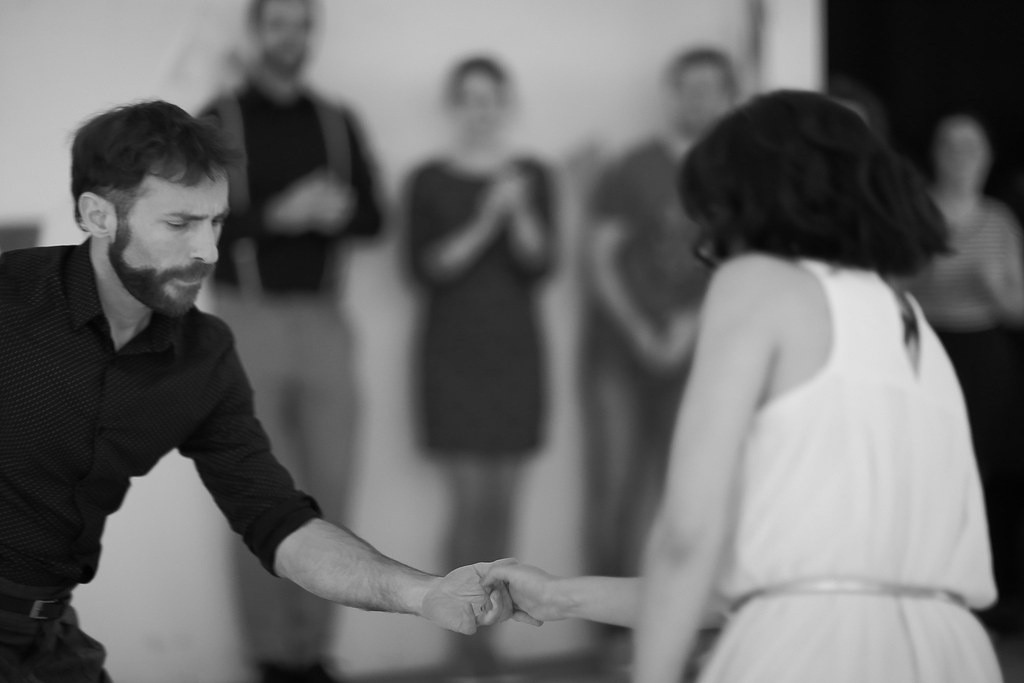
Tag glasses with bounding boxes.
[690,224,716,271]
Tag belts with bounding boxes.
[0,596,72,619]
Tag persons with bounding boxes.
[0,98,544,681]
[594,48,735,571]
[407,56,556,676]
[920,117,1024,335]
[481,91,1006,681]
[198,0,390,683]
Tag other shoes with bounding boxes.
[252,658,344,683]
